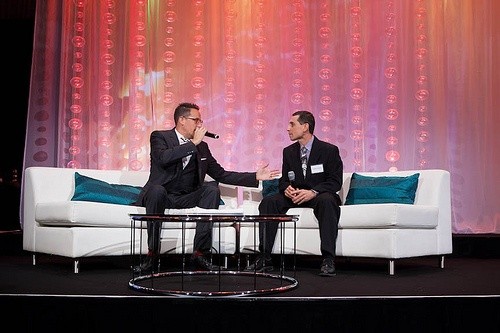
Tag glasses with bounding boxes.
[185,117,203,125]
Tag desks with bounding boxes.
[126,213,301,296]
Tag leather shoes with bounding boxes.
[133,254,168,274]
[318,256,336,276]
[188,255,225,271]
[244,255,273,272]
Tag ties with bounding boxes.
[300,147,308,180]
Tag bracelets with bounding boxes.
[311,189,317,195]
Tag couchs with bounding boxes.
[20,166,454,277]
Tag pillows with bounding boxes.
[344,172,419,204]
[71,172,144,207]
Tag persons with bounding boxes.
[134,103,280,274]
[243,110,343,276]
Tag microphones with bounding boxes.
[288,170,299,196]
[205,132,219,139]
